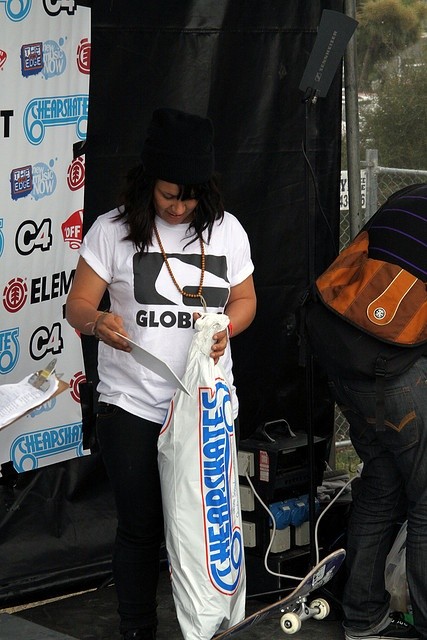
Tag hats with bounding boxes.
[131,110,215,192]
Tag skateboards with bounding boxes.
[211,548,347,640]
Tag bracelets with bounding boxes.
[228,323,232,336]
[84,311,110,342]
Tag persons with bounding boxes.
[65,107,258,640]
[303,181,427,640]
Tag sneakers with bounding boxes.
[346,608,426,639]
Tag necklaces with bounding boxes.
[153,221,206,313]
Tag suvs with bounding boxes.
[342,94,387,145]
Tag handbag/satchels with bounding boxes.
[284,228,427,380]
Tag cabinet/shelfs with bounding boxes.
[237,417,327,603]
